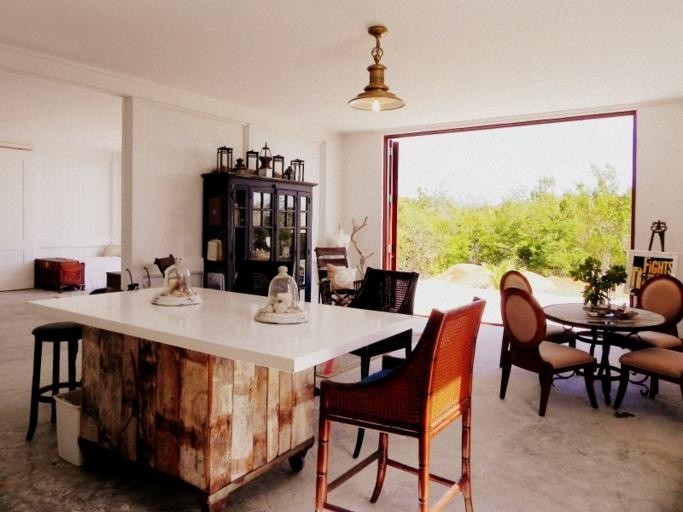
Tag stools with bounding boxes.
[24,321,81,442]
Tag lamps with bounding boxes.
[345,26,406,112]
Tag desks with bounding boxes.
[33,256,84,294]
[540,301,667,407]
[24,285,420,511]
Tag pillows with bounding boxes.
[325,263,358,292]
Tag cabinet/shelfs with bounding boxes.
[200,171,319,303]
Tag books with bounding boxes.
[202,194,228,291]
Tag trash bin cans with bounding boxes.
[53,390,86,466]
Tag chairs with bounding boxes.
[498,286,599,416]
[498,269,576,369]
[611,346,682,410]
[312,245,363,307]
[346,266,419,459]
[610,273,683,395]
[316,295,487,511]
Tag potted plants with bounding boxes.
[564,254,628,317]
